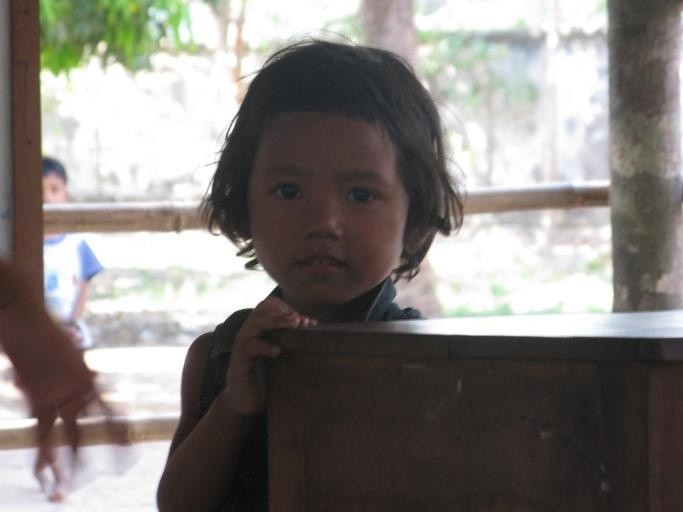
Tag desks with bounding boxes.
[268,312,682,512]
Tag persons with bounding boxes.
[156,42,464,511]
[32,157,103,502]
